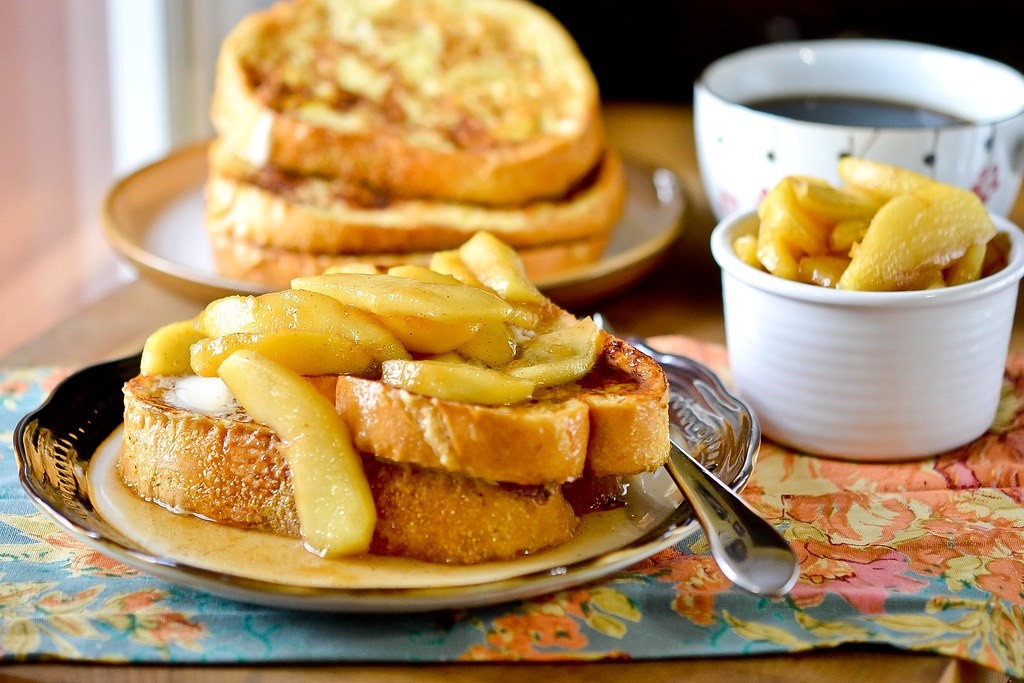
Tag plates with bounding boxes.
[11,331,760,613]
[96,137,688,309]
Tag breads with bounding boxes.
[122,296,672,564]
[200,1,623,256]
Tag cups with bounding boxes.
[693,38,1024,222]
[710,208,1024,463]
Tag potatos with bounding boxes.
[141,231,604,555]
[735,155,998,292]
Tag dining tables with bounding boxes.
[0,95,1024,683]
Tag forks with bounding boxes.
[577,313,798,599]
